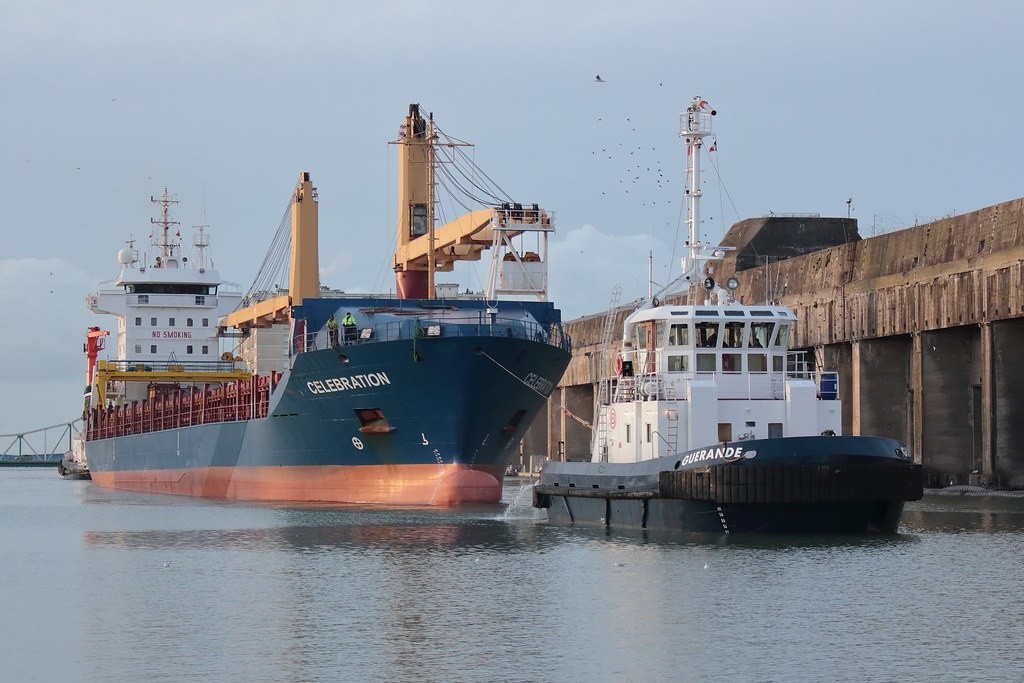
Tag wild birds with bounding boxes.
[110,96,120,103]
[47,271,57,296]
[148,176,150,179]
[591,75,720,269]
[24,158,32,165]
[77,167,80,170]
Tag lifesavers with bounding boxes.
[615,355,623,375]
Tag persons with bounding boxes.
[750,338,763,348]
[705,328,729,348]
[327,315,339,347]
[342,312,356,345]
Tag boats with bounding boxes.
[81,103,575,510]
[531,94,927,538]
[57,429,92,481]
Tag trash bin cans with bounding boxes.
[821,374,838,400]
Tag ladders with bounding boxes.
[597,284,623,463]
